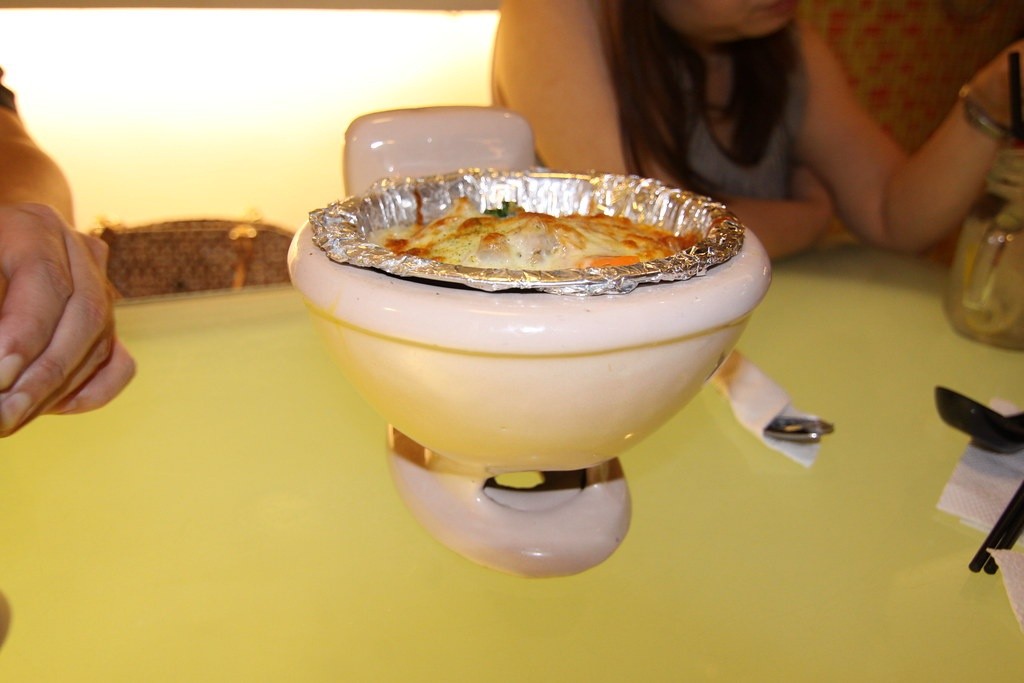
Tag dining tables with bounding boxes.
[0,247,1024,683]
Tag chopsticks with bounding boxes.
[968,483,1024,575]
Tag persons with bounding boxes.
[0,64,134,438]
[490,0,1024,259]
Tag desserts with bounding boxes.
[359,197,691,275]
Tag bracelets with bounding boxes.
[966,101,1010,141]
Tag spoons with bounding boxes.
[934,386,1024,455]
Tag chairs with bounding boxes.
[92,219,295,300]
